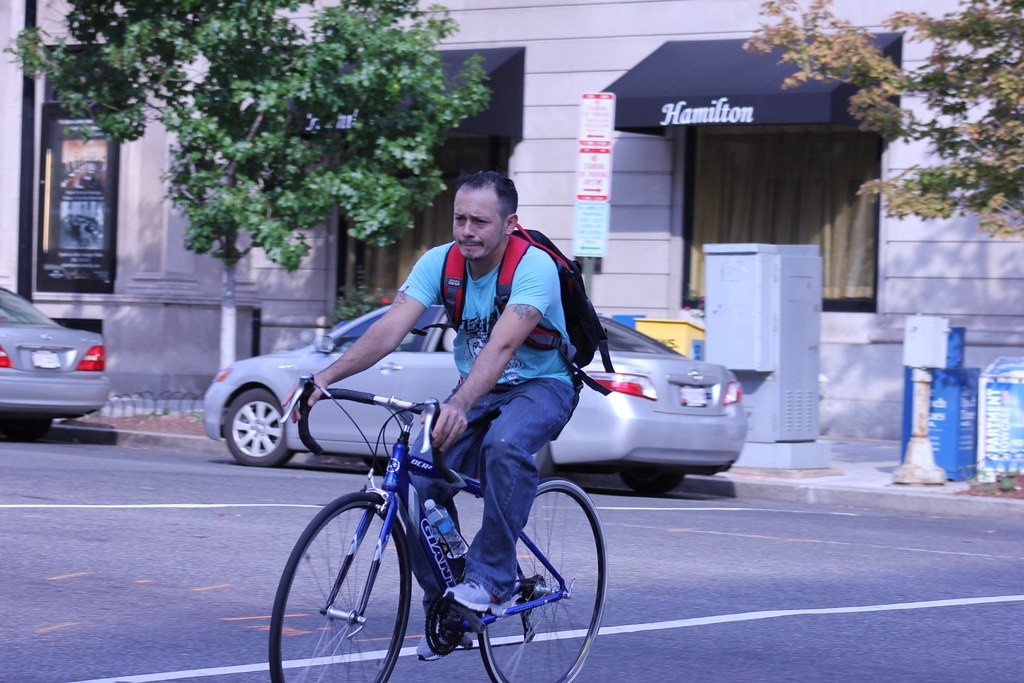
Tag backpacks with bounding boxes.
[441,228,615,396]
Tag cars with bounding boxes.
[0,287,108,440]
[204,295,747,495]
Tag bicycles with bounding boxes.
[267,374,610,683]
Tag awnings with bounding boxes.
[598,33,903,137]
[287,44,526,139]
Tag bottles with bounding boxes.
[424,499,465,559]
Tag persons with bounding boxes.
[280,169,581,661]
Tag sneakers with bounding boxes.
[416,619,448,661]
[442,578,509,617]
